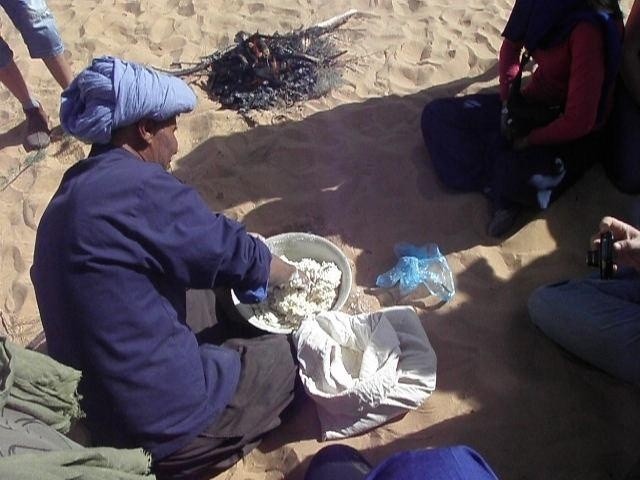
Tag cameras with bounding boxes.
[585,232,616,279]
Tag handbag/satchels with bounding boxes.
[506,86,564,134]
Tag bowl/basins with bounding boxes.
[226,230,351,335]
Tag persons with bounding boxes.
[0,0,75,149]
[525,216,640,389]
[31,55,311,479]
[420,0,625,240]
[307,444,498,479]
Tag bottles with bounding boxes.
[599,234,614,278]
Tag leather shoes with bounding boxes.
[490,204,519,238]
